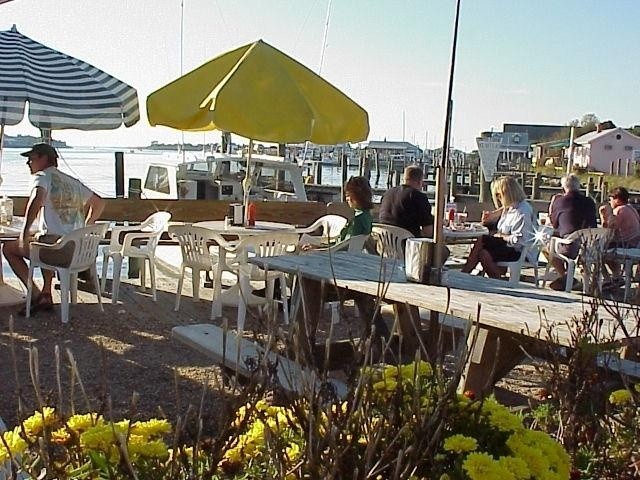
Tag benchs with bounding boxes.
[416,309,639,390]
[168,321,371,417]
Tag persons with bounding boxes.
[460,175,533,278]
[2,142,105,318]
[378,164,450,268]
[251,174,372,300]
[598,188,640,291]
[542,172,598,290]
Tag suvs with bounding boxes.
[498,161,516,171]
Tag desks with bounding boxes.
[443,223,489,269]
[246,250,639,400]
[1,215,38,308]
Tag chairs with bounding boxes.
[99,211,172,305]
[168,213,371,334]
[25,222,109,323]
[371,223,415,261]
[496,210,640,303]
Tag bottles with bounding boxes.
[0,193,14,227]
[224,215,230,231]
[247,201,256,227]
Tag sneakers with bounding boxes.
[601,276,626,292]
[18,287,54,318]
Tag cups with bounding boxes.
[540,214,547,225]
[457,212,468,223]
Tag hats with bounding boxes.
[20,143,60,158]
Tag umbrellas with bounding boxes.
[145,39,370,224]
[0,22,139,133]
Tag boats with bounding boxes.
[126,143,326,276]
[215,127,359,170]
